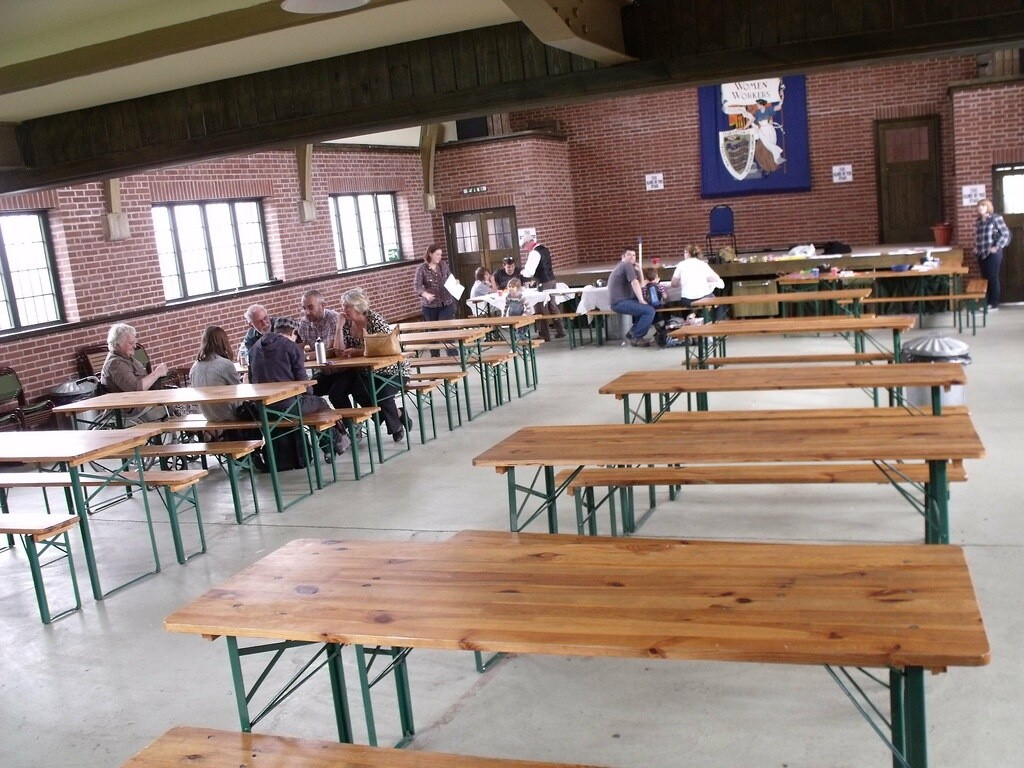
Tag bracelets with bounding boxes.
[151,372,157,380]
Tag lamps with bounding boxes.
[281,0,369,14]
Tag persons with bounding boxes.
[494,257,527,290]
[189,326,264,471]
[102,323,223,443]
[469,266,504,342]
[503,278,534,356]
[643,268,671,346]
[332,289,412,442]
[413,245,458,358]
[519,235,566,341]
[973,199,1009,312]
[237,304,277,365]
[607,247,655,346]
[247,318,350,464]
[672,245,728,339]
[297,291,362,444]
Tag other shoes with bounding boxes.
[186,455,199,461]
[555,332,565,338]
[325,452,333,463]
[393,426,404,442]
[631,337,649,347]
[980,304,1000,313]
[626,332,633,340]
[398,408,412,431]
[335,434,351,455]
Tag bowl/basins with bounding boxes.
[841,271,853,276]
[891,265,910,271]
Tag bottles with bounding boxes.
[239,342,249,368]
[597,279,604,287]
[315,337,326,364]
[304,345,311,361]
[647,256,651,264]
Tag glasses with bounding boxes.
[503,257,513,261]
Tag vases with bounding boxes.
[930,224,953,247]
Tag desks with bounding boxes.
[399,327,491,421]
[0,427,164,600]
[51,378,318,512]
[690,288,873,358]
[598,361,969,412]
[165,540,991,768]
[669,313,918,414]
[234,354,402,465]
[778,265,970,338]
[398,315,542,399]
[469,282,605,348]
[471,413,986,545]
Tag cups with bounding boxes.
[498,292,502,297]
[934,259,940,267]
[813,270,819,278]
[326,349,335,358]
[525,282,543,292]
[831,268,837,276]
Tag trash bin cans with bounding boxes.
[48,376,100,430]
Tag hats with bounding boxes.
[519,235,532,248]
[274,317,304,343]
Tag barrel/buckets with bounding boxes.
[930,224,953,245]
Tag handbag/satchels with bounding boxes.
[237,402,258,420]
[261,427,312,469]
[993,229,1012,247]
[363,325,400,356]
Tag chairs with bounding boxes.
[706,203,737,255]
[81,344,161,392]
[0,367,56,432]
[132,343,191,388]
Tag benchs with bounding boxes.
[1,275,992,767]
